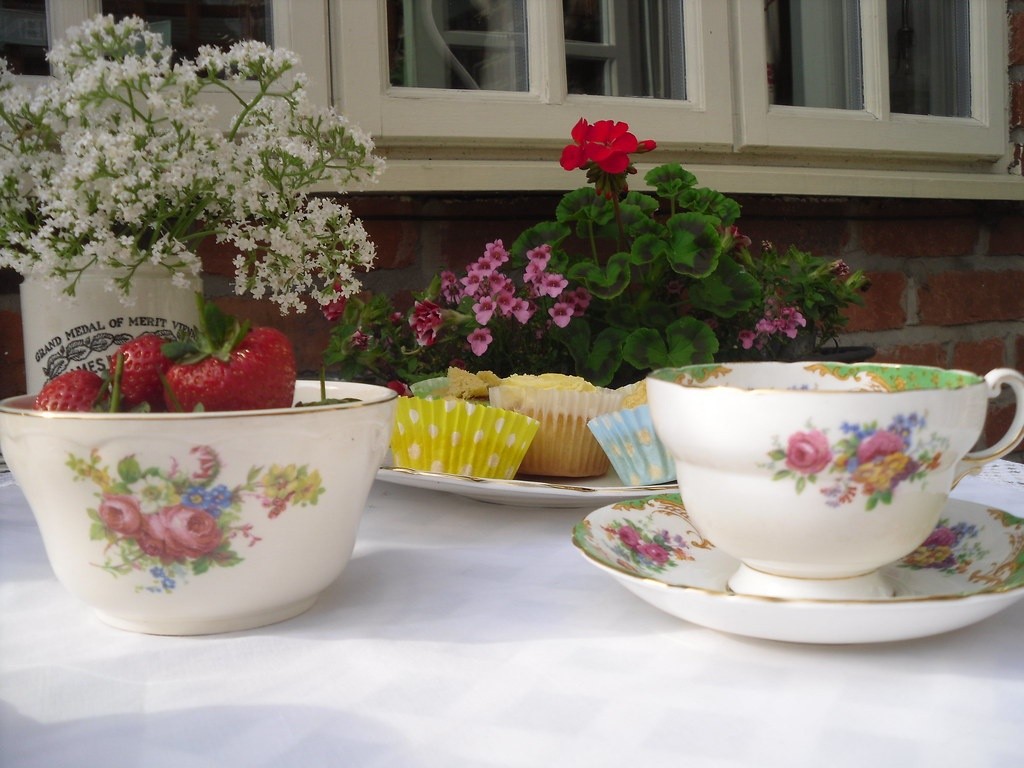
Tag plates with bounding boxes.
[375,447,683,507]
[571,491,1024,645]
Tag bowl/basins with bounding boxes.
[0,381,401,637]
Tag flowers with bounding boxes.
[0,15,380,319]
[325,117,872,397]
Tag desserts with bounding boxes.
[389,366,677,485]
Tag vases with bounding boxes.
[19,258,207,401]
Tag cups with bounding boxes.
[645,361,1024,599]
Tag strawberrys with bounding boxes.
[33,289,366,412]
